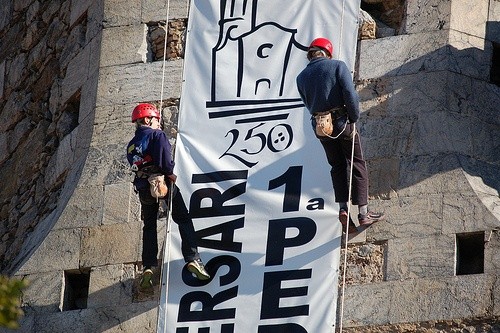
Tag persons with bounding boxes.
[295,38,385,234]
[127,103,210,290]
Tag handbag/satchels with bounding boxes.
[148,174,168,202]
[315,113,333,136]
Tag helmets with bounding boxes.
[310,38,333,59]
[132,103,160,123]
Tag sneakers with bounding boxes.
[358,207,385,225]
[339,208,358,234]
[140,266,154,294]
[188,259,210,280]
